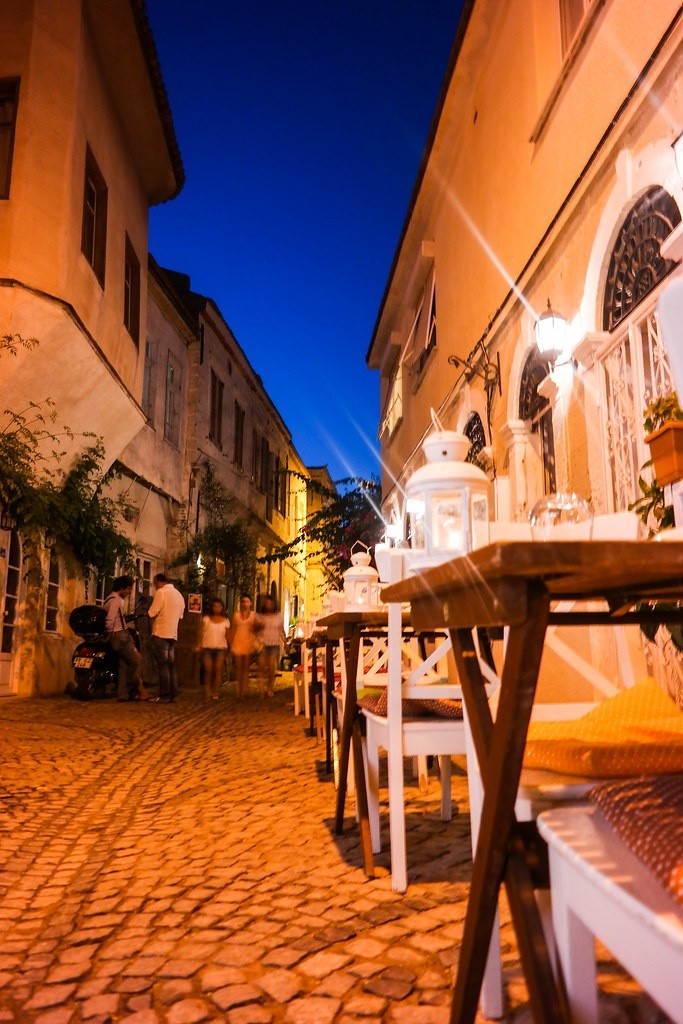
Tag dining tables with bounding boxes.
[312,629,448,773]
[379,542,683,1024]
[316,613,503,876]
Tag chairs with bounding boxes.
[280,511,683,1024]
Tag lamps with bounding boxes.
[534,297,572,372]
[405,406,491,574]
[343,539,379,613]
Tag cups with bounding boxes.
[529,493,594,540]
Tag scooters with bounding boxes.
[69,596,151,700]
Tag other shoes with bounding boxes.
[147,695,171,705]
[203,694,219,705]
[236,692,247,702]
[119,695,136,702]
[258,691,264,701]
[268,692,275,698]
[139,696,161,704]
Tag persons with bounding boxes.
[257,594,287,700]
[100,575,161,701]
[146,572,185,702]
[230,593,259,698]
[195,596,230,704]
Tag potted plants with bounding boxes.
[643,392,683,488]
